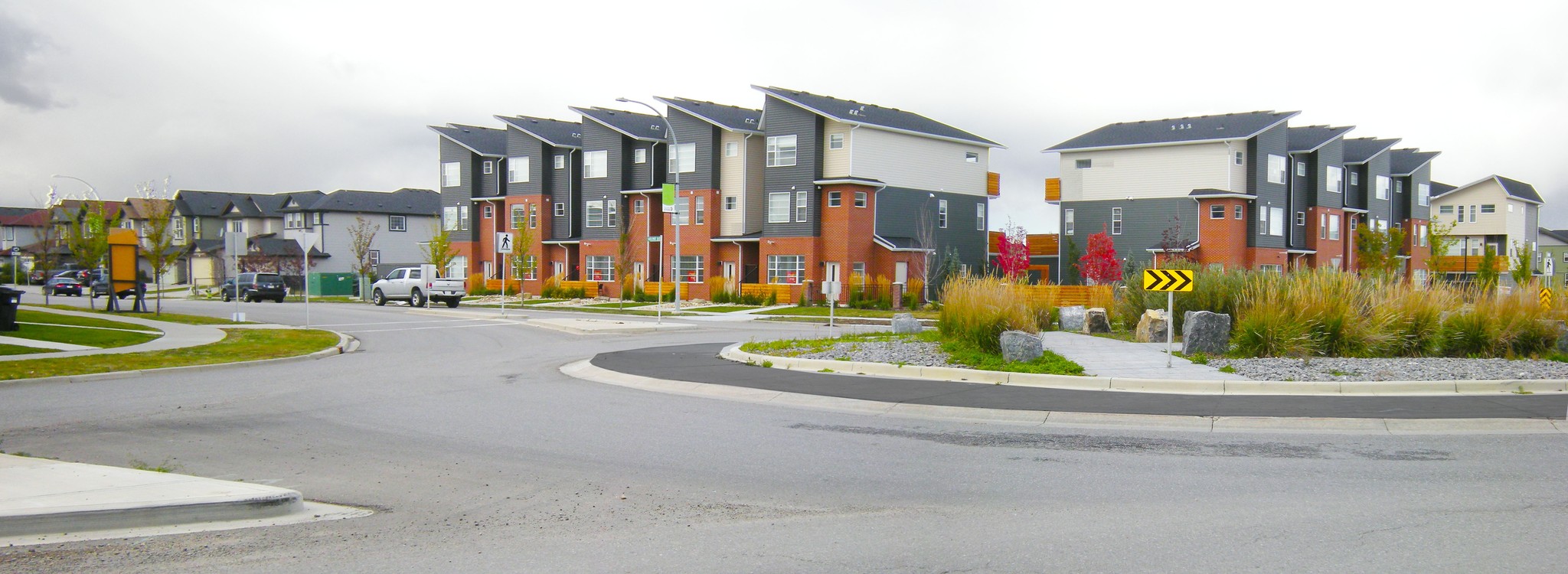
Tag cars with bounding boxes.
[91,274,146,300]
[28,261,108,288]
[41,277,82,297]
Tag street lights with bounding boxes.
[1464,235,1470,292]
[615,96,681,313]
[51,173,104,278]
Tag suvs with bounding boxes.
[220,271,287,303]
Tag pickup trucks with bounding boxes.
[371,267,467,308]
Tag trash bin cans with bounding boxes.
[0,286,26,331]
